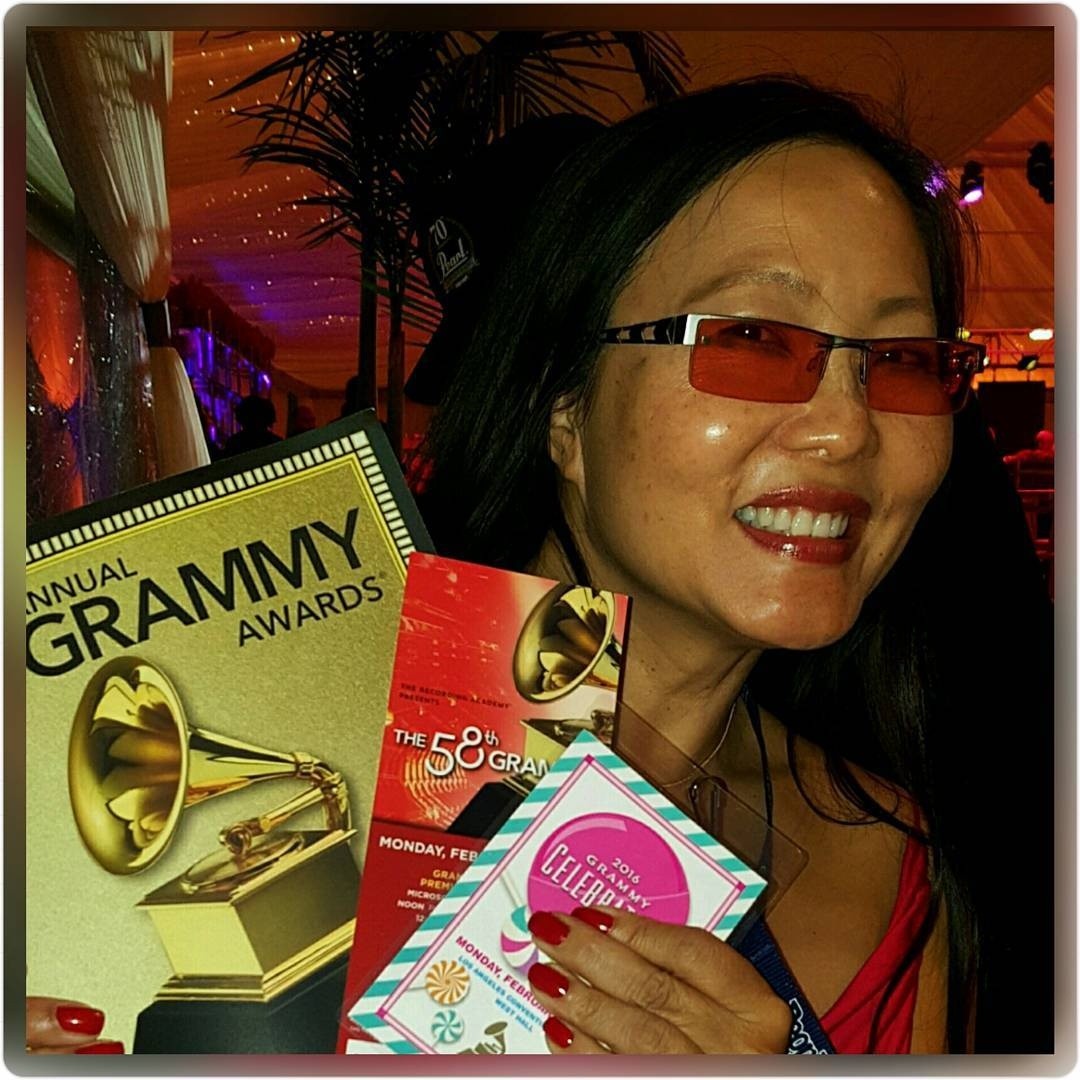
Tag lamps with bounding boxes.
[1018,355,1040,370]
[960,160,984,203]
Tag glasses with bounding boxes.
[581,312,986,416]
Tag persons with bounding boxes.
[25,73,1055,1052]
[216,375,396,461]
